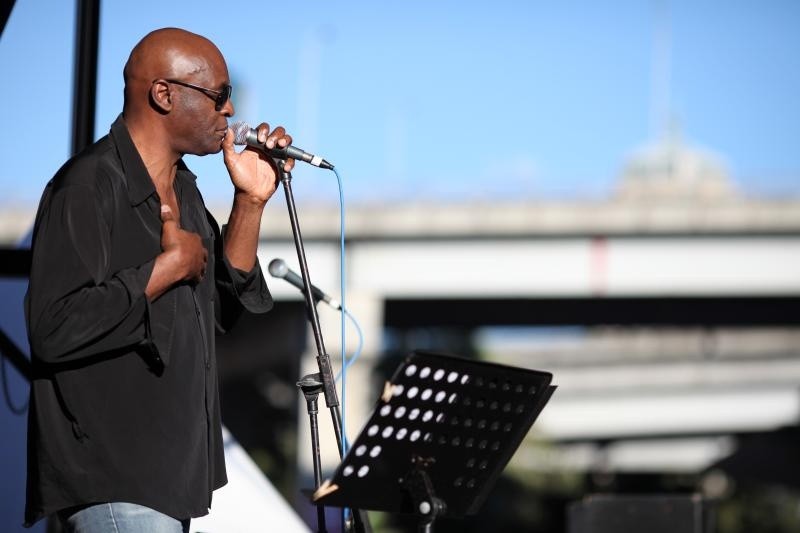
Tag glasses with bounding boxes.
[152,77,233,111]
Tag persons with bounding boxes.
[23,28,295,533]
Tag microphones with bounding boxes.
[229,121,335,171]
[269,259,342,312]
